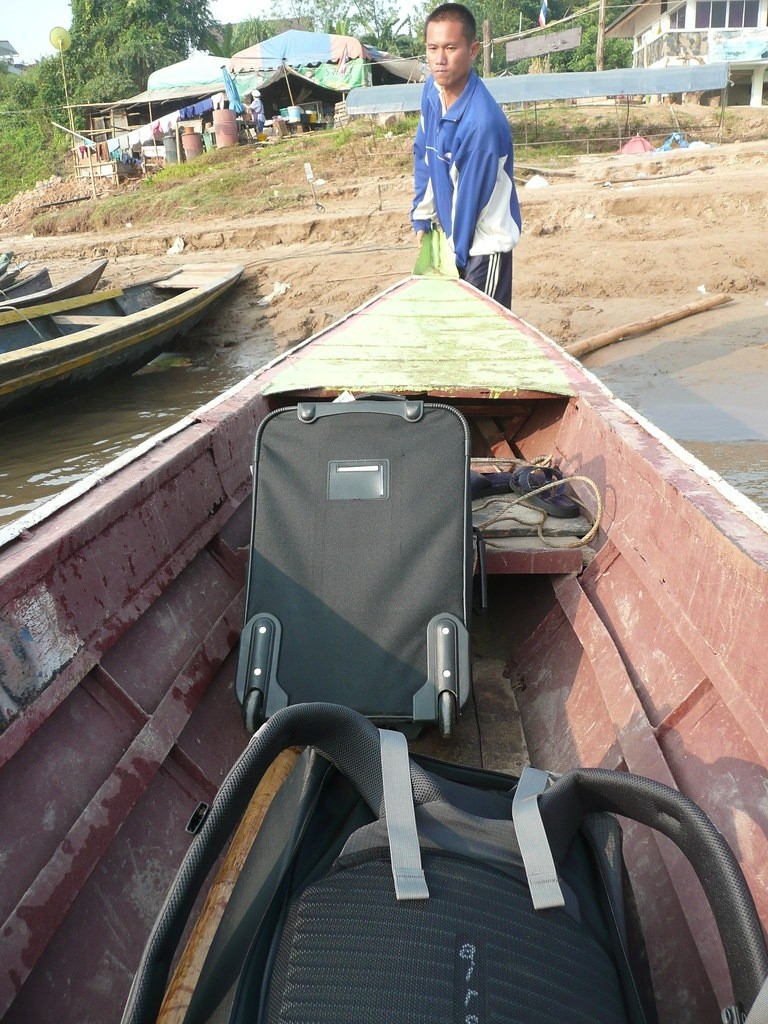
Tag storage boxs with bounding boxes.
[278,107,289,116]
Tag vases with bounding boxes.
[707,96,720,106]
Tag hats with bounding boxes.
[252,90,260,96]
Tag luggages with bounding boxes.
[235,394,485,740]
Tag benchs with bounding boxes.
[238,456,581,575]
[54,314,125,337]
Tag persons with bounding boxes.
[242,90,265,131]
[411,3,521,308]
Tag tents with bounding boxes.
[148,56,230,160]
[228,29,426,110]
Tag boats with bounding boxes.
[0,251,245,421]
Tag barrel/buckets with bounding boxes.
[212,108,238,149]
[181,131,204,163]
[162,136,184,164]
[286,106,301,122]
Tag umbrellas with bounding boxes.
[220,65,245,113]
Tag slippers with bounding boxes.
[509,465,580,518]
[470,469,511,500]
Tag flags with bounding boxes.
[539,0,548,26]
